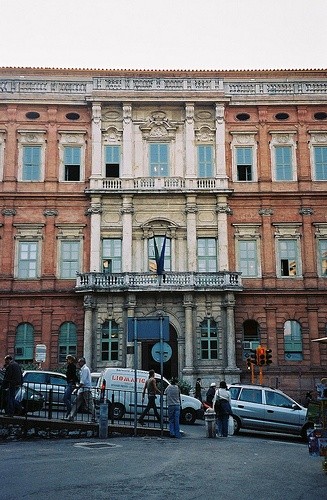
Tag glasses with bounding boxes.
[65,359,68,361]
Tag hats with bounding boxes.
[211,382,216,386]
[219,381,227,388]
[169,377,178,383]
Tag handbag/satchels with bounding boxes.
[15,387,26,402]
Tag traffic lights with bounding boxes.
[250,348,258,366]
[264,346,273,366]
[257,345,266,366]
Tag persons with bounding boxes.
[205,383,216,408]
[213,381,230,439]
[138,370,162,425]
[195,378,202,403]
[164,378,181,438]
[1,355,24,413]
[61,355,96,422]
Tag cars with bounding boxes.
[212,381,315,441]
[21,369,82,405]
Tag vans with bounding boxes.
[94,367,205,426]
[90,372,102,400]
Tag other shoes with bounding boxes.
[2,414,12,418]
[216,433,223,438]
[137,421,146,425]
[62,416,74,421]
[223,434,227,438]
[22,407,28,417]
[88,419,98,424]
